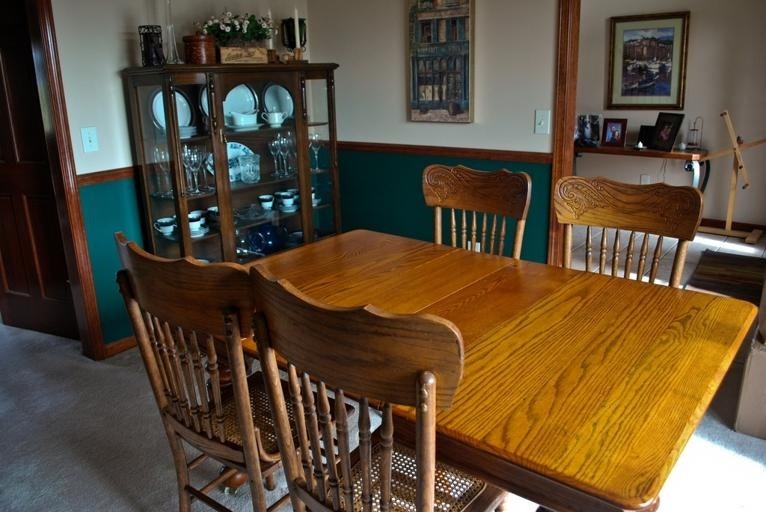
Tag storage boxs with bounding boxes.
[734,329,766,439]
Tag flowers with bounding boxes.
[194,10,276,44]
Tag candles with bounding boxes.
[294,8,301,61]
[267,8,276,63]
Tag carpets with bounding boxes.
[685,248,766,305]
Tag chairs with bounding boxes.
[554,175,705,289]
[249,265,507,512]
[423,163,532,260]
[114,229,355,511]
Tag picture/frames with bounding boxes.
[648,112,685,152]
[406,0,475,123]
[601,117,627,147]
[578,114,599,142]
[607,11,691,110]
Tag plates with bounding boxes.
[147,85,196,129]
[197,82,260,128]
[261,82,295,119]
[233,125,260,132]
[205,141,257,184]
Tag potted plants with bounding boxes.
[217,40,268,64]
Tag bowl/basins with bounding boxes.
[228,110,259,128]
[176,125,195,138]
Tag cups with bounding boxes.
[186,143,206,195]
[259,193,274,212]
[310,133,322,172]
[148,143,163,196]
[281,191,295,208]
[275,133,284,174]
[239,153,261,184]
[310,186,315,202]
[287,189,300,202]
[307,132,312,147]
[261,106,290,129]
[278,135,292,177]
[157,146,172,197]
[153,217,175,238]
[267,139,280,177]
[274,190,284,204]
[186,213,203,234]
[198,145,214,192]
[181,143,195,191]
[206,206,220,224]
[287,129,297,176]
[189,209,207,228]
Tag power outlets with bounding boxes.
[81,126,100,154]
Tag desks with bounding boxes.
[194,229,760,512]
[574,146,710,194]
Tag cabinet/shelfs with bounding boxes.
[120,63,342,264]
